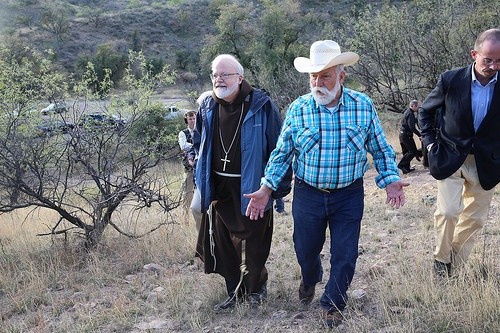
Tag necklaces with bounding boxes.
[219,104,244,171]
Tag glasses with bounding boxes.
[209,73,239,79]
[476,51,500,65]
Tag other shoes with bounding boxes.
[403,168,415,174]
[247,281,267,305]
[433,260,451,277]
[397,164,408,173]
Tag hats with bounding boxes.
[294,40,359,73]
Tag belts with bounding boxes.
[295,176,363,193]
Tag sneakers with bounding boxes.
[298,279,316,305]
[323,304,342,328]
[218,294,244,310]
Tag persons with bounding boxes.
[194,54,293,311]
[419,28,500,278]
[244,40,411,328]
[416,106,429,168]
[397,100,419,174]
[178,112,198,212]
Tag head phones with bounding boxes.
[184,111,197,124]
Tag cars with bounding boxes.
[40,102,70,116]
[78,113,127,128]
[164,106,189,120]
[36,119,74,137]
